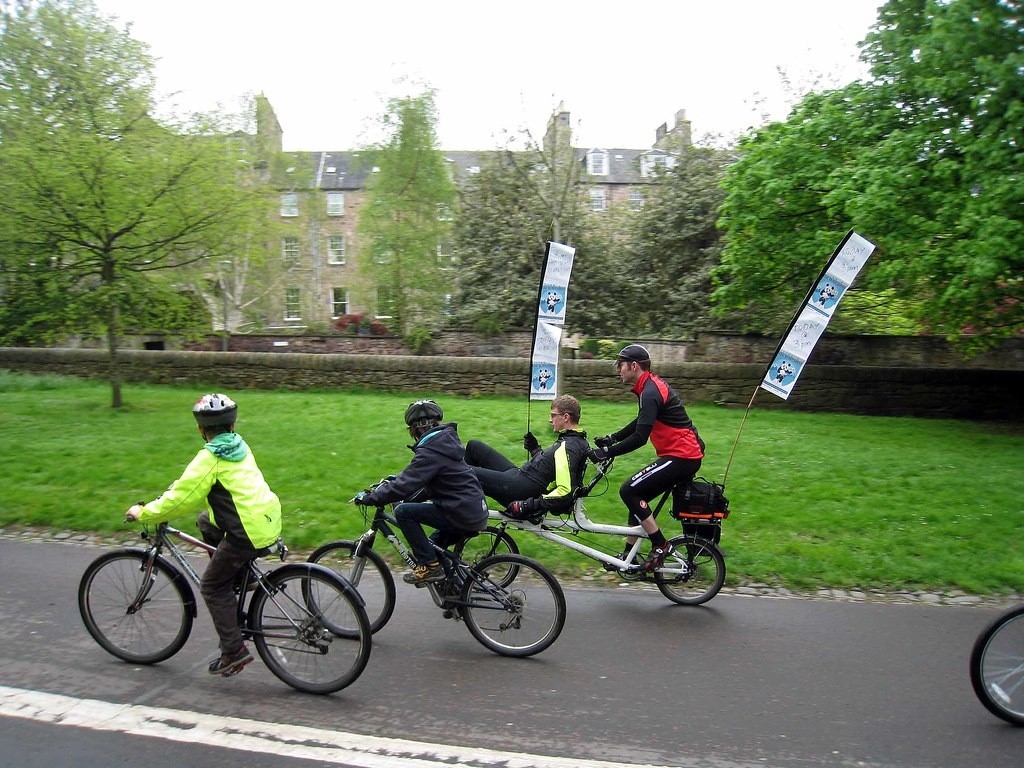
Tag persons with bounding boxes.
[354,398,490,620]
[124,393,283,673]
[587,346,705,570]
[465,394,588,518]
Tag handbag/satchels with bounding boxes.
[672,477,727,519]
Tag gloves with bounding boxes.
[587,448,608,463]
[383,474,397,483]
[523,432,537,451]
[354,491,371,505]
[507,497,534,518]
[593,437,611,448]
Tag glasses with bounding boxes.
[550,412,564,418]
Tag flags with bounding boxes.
[760,232,876,400]
[530,242,575,399]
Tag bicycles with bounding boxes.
[76,499,373,697]
[300,471,567,659]
[968,602,1024,728]
[452,433,732,608]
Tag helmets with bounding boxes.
[404,399,445,428]
[192,392,239,426]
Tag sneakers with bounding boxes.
[207,646,253,674]
[443,598,476,619]
[233,571,260,589]
[637,540,673,572]
[602,550,637,571]
[403,563,447,583]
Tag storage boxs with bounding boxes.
[682,517,720,555]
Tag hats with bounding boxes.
[613,344,649,365]
